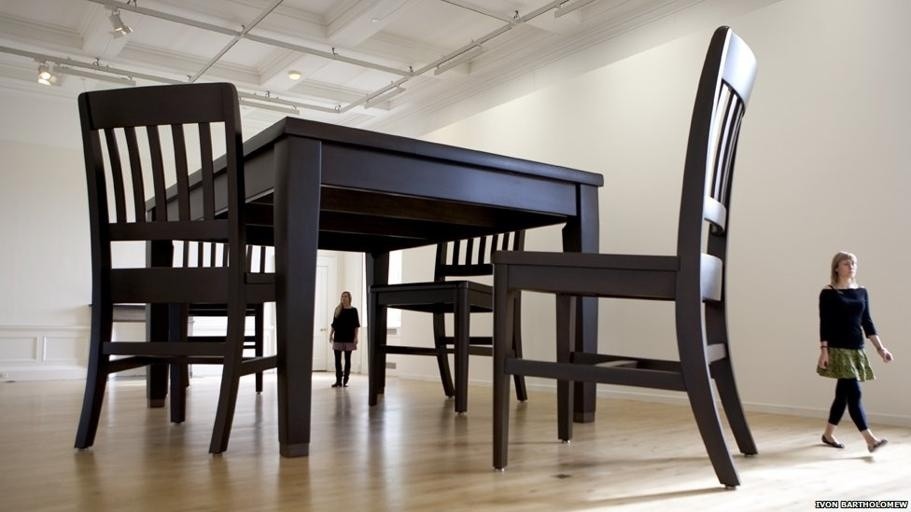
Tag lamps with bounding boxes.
[37,60,53,86]
[109,8,131,38]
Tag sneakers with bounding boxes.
[332,378,350,389]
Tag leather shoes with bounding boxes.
[868,437,889,453]
[820,434,846,450]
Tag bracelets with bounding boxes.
[821,346,829,348]
[879,346,888,356]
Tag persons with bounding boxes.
[329,291,360,388]
[815,251,895,452]
